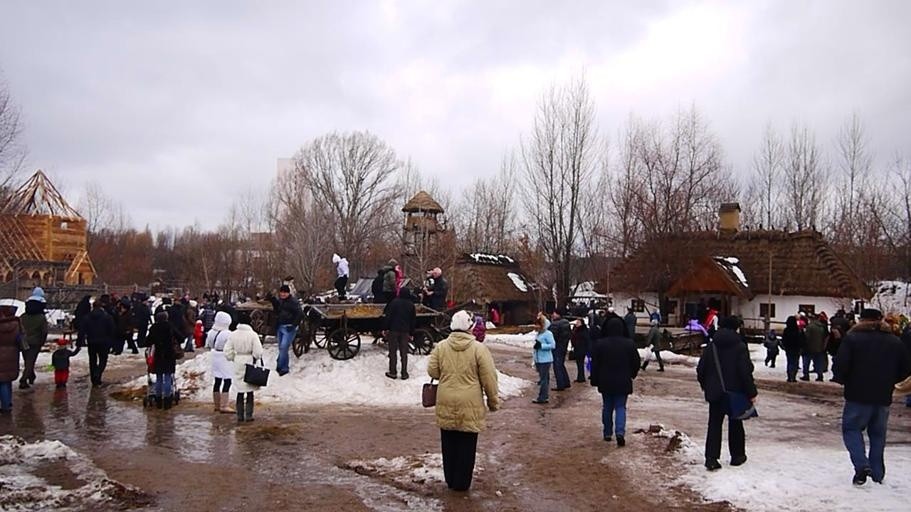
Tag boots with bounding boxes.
[219,392,236,413]
[155,396,162,409]
[245,402,254,422]
[163,397,169,410]
[213,391,221,411]
[236,402,244,421]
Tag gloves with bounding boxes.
[533,339,540,349]
[286,325,293,332]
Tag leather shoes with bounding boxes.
[705,459,721,469]
[19,383,30,389]
[401,373,408,379]
[729,455,747,465]
[29,375,35,384]
[604,435,611,441]
[385,372,396,379]
[616,435,624,446]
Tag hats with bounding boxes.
[450,310,477,333]
[388,259,398,266]
[57,339,65,346]
[332,254,340,263]
[651,319,658,325]
[857,309,882,320]
[280,285,289,293]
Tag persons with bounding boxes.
[830,308,911,489]
[372,259,450,312]
[384,287,418,380]
[531,308,665,404]
[224,314,264,422]
[138,311,177,409]
[270,285,303,376]
[0,286,237,412]
[590,314,640,446]
[333,252,350,300]
[205,310,237,414]
[428,310,501,493]
[696,316,759,471]
[702,308,911,409]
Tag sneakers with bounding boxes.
[853,468,872,485]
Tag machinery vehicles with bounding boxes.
[292,303,440,359]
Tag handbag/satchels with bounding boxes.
[243,356,269,386]
[17,318,30,351]
[172,336,183,359]
[724,390,759,421]
[421,378,437,407]
[568,347,575,360]
